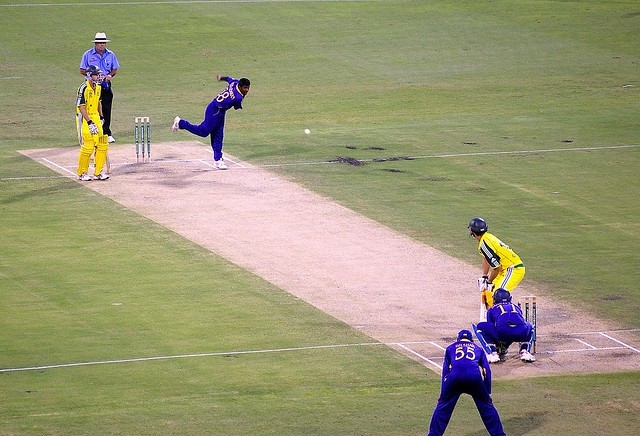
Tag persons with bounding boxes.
[473,288,536,363]
[172,75,250,170]
[467,217,526,314]
[80,32,119,142]
[427,330,505,436]
[76,65,110,181]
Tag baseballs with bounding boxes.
[305,129,310,135]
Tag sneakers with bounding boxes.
[77,173,91,181]
[520,350,535,362]
[171,116,180,132]
[487,353,501,363]
[94,172,109,180]
[216,159,227,170]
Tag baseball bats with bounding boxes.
[480,292,487,322]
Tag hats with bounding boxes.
[91,32,111,43]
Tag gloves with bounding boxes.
[88,121,98,135]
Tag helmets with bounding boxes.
[469,218,487,234]
[85,65,102,76]
[493,288,512,302]
[458,330,473,340]
